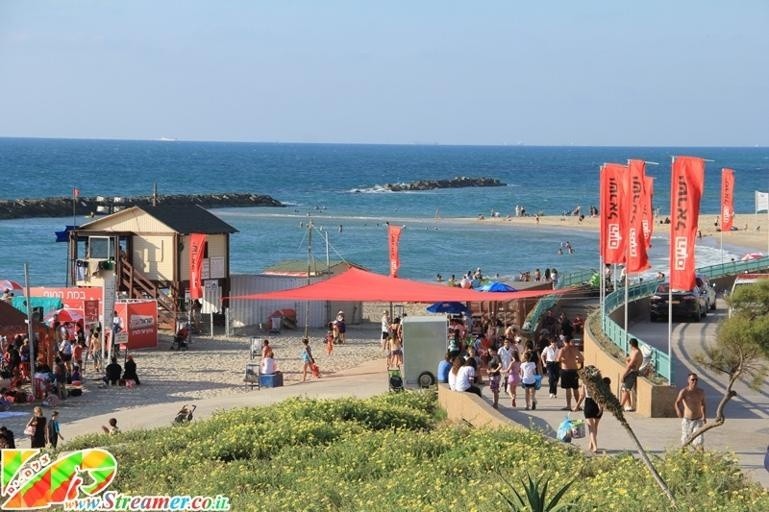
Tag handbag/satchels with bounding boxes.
[23,425,36,437]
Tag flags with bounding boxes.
[74,188,81,198]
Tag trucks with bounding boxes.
[722,268,768,322]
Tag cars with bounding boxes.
[763,445,769,473]
[646,279,711,323]
[690,269,720,310]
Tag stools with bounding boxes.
[259,370,283,388]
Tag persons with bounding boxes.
[674,373,707,454]
[325,310,345,355]
[617,338,644,411]
[1,244,202,448]
[380,267,608,449]
[338,204,719,256]
[263,340,273,360]
[300,338,321,382]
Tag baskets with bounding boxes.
[570,419,585,438]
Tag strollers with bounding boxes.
[173,403,198,423]
[387,364,404,394]
